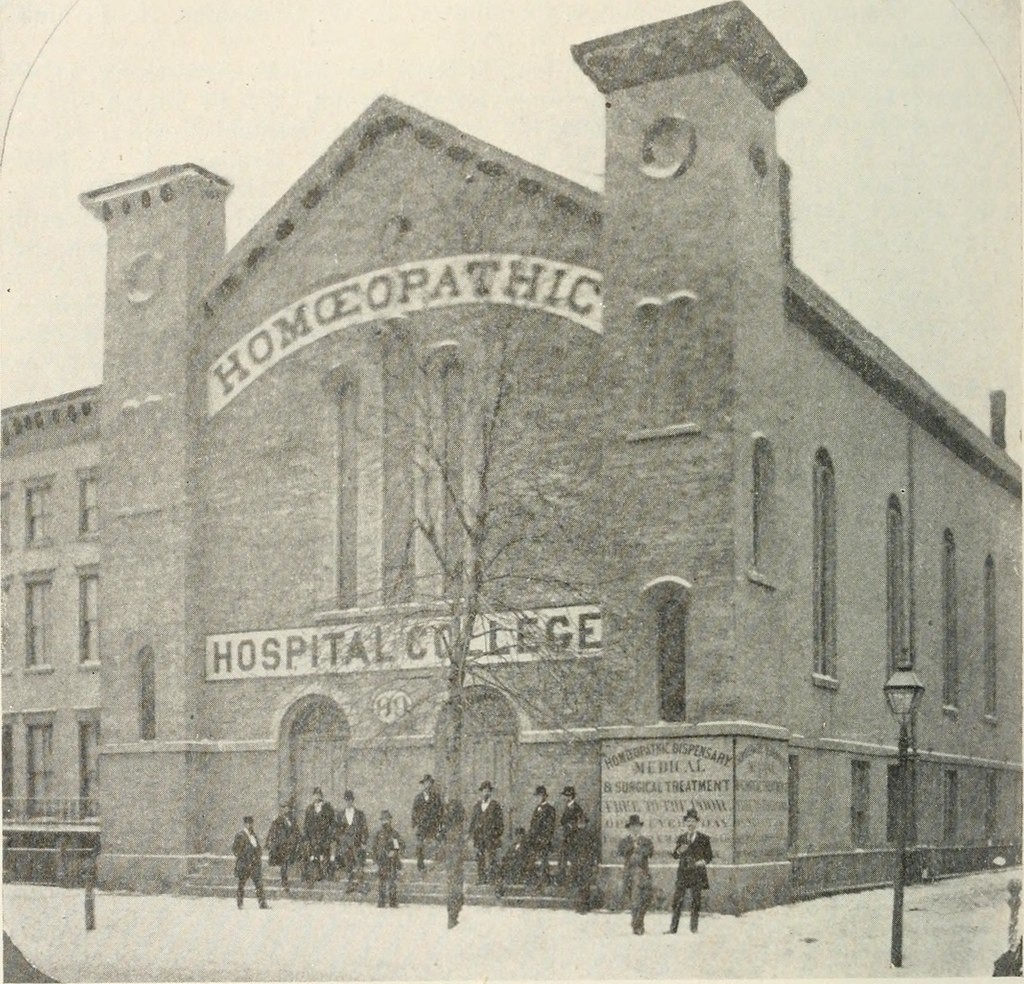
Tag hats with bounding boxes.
[243,816,253,823]
[313,788,322,795]
[513,828,526,836]
[343,790,354,800]
[534,786,548,797]
[562,787,576,795]
[684,809,700,821]
[626,815,644,828]
[420,774,434,784]
[479,781,494,790]
[379,810,392,819]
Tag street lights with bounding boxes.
[882,647,927,969]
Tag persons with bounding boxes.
[264,803,315,891]
[491,782,606,912]
[662,808,712,935]
[469,781,504,885]
[618,815,653,935]
[410,773,448,871]
[302,785,369,891]
[231,815,272,910]
[371,810,405,909]
